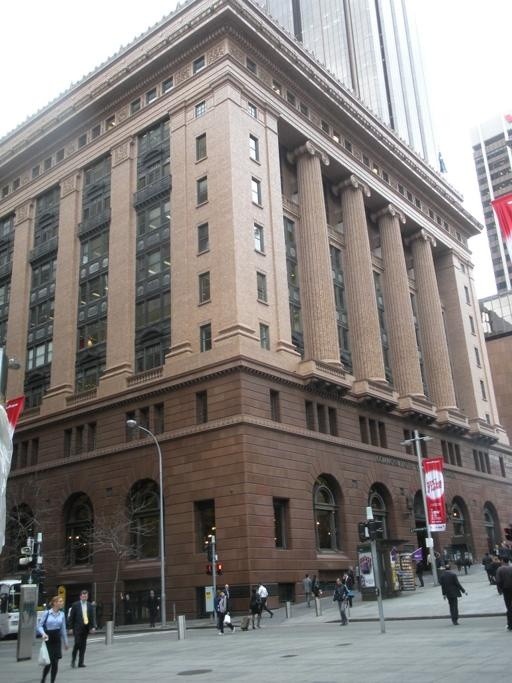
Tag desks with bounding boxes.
[400,429,444,586]
[126,420,168,627]
[202,526,223,626]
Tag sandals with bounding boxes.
[1,579,47,638]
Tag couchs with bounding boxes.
[218,563,223,575]
[207,564,212,574]
[503,527,511,540]
[376,520,383,537]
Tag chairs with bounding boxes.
[218,626,236,636]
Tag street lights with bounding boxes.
[35,610,50,637]
[223,614,231,625]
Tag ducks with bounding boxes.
[240,616,251,631]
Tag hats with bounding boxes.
[455,551,475,566]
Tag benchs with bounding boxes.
[422,456,448,532]
[491,191,512,261]
[4,394,26,440]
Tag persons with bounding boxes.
[68,590,97,668]
[37,595,68,683]
[8,602,17,612]
[214,590,222,629]
[333,566,355,626]
[217,592,236,635]
[67,599,104,634]
[303,574,313,608]
[312,574,320,599]
[249,590,262,629]
[223,584,232,613]
[415,540,512,632]
[146,589,160,627]
[257,582,274,619]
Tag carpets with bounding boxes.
[81,600,89,624]
